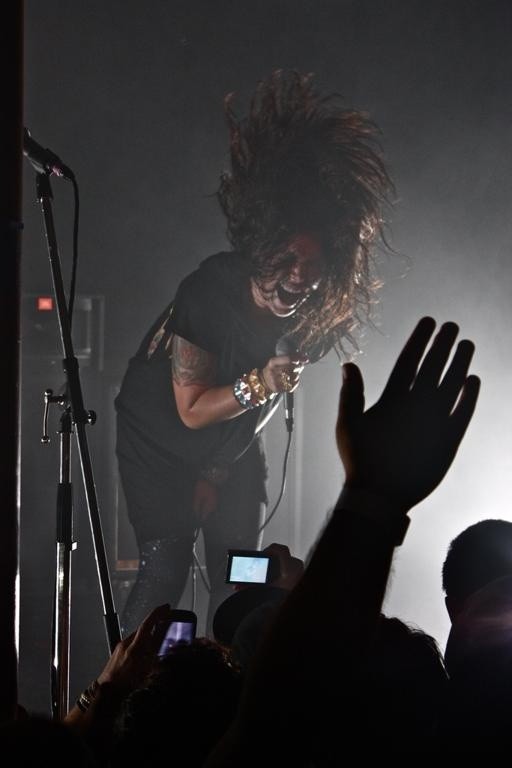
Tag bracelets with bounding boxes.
[335,484,410,544]
[232,368,276,412]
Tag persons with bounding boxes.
[0,545,450,767]
[115,72,401,645]
[204,315,511,767]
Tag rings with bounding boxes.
[277,368,293,394]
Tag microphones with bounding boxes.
[22,129,74,180]
[276,337,296,433]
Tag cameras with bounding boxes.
[225,548,277,585]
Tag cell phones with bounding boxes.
[151,610,197,663]
